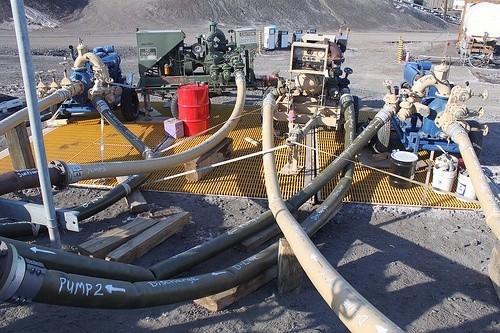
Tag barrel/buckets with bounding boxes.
[435,154,459,166]
[432,165,457,194]
[177,83,210,138]
[389,151,418,188]
[455,170,477,202]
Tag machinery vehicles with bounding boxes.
[49,38,142,121]
